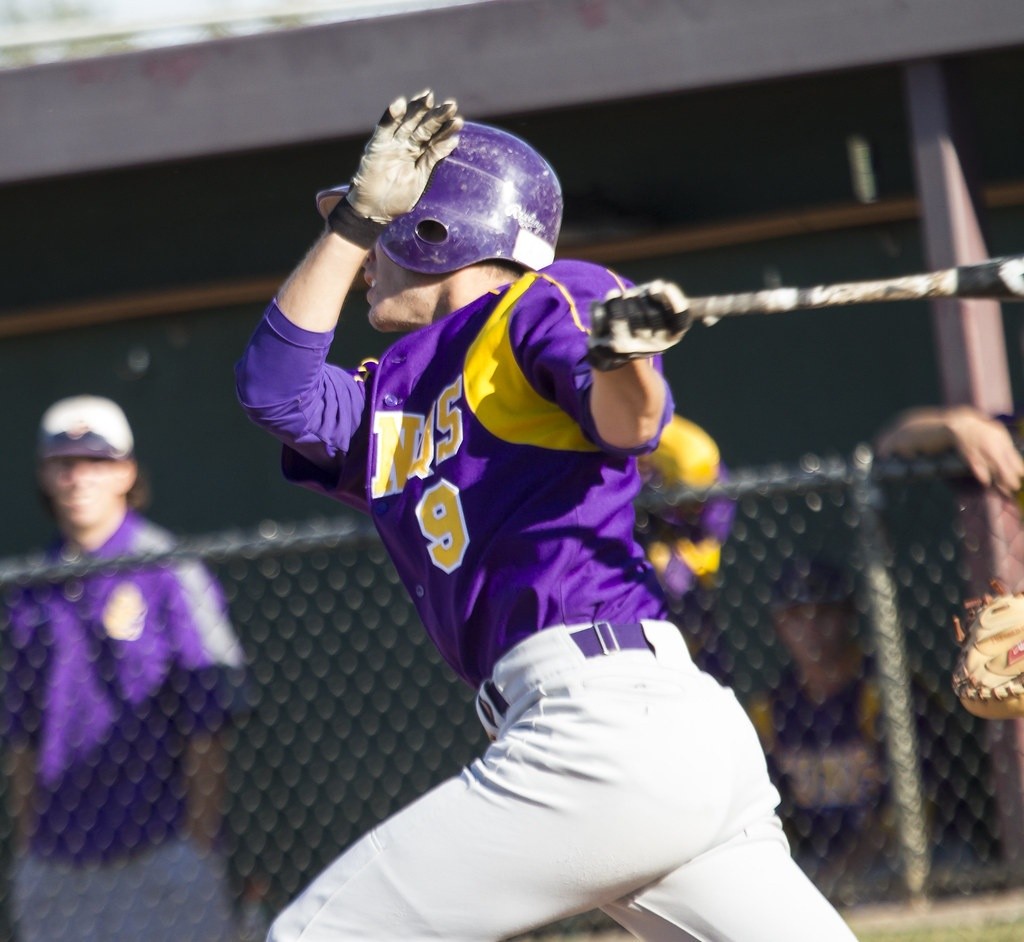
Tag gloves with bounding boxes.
[582,279,692,372]
[326,90,463,250]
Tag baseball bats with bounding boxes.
[593,257,1024,332]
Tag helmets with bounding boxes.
[316,123,563,275]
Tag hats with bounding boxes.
[38,393,133,459]
[766,556,851,613]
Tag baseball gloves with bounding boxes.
[952,582,1024,720]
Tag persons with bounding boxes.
[630,407,1024,868]
[246,86,862,942]
[0,394,248,942]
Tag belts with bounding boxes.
[478,621,652,740]
